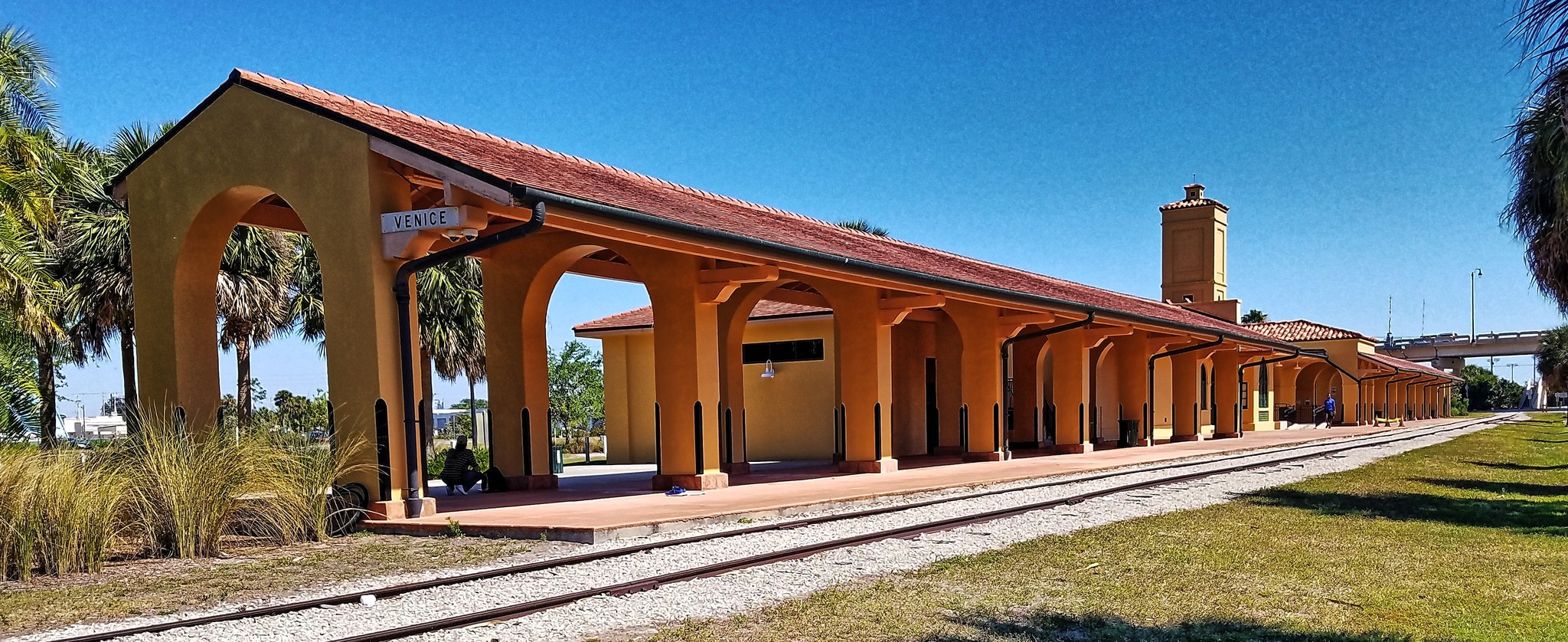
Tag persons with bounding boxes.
[1324,393,1336,429]
[439,435,483,495]
[433,426,443,437]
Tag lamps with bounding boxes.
[1276,361,1282,367]
[1294,362,1301,370]
[761,359,775,379]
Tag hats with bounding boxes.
[457,435,466,444]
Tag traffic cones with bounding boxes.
[1384,414,1390,427]
[1373,415,1380,427]
[1398,414,1406,427]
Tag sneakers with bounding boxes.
[665,485,688,496]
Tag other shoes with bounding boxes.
[455,485,468,495]
[1326,425,1331,429]
[445,486,456,497]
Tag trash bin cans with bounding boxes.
[1374,411,1383,423]
[552,445,564,473]
[1408,410,1412,420]
[1117,420,1140,447]
[1431,410,1435,419]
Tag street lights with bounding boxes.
[1471,267,1483,344]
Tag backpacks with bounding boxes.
[482,466,505,493]
